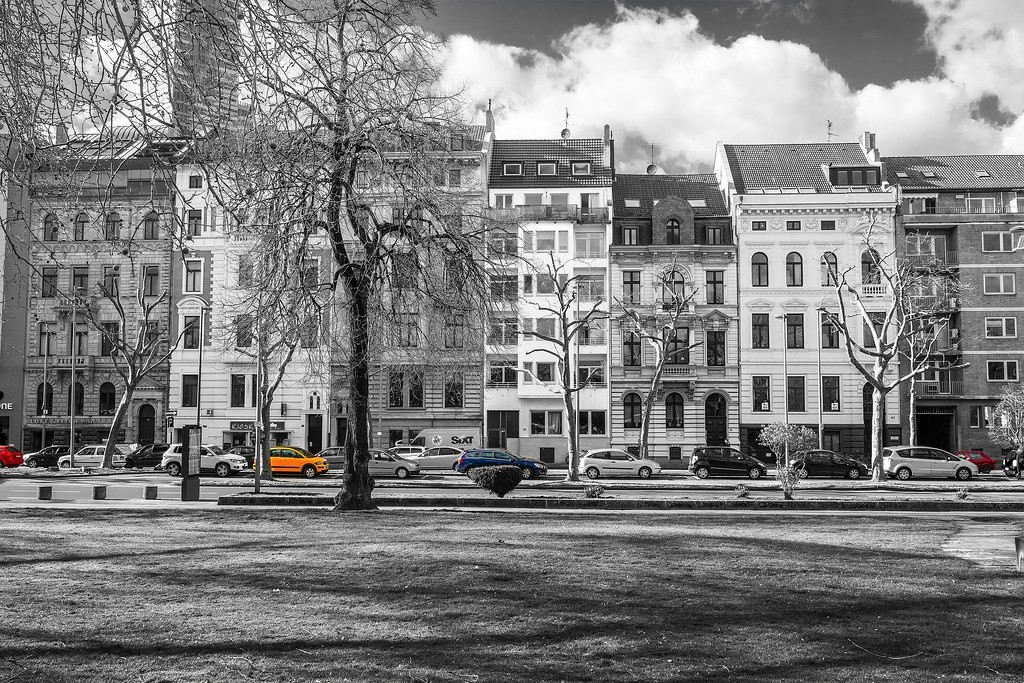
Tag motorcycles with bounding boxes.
[565,449,583,465]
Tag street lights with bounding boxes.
[196,306,212,424]
[775,313,790,469]
[69,285,85,469]
[815,307,825,449]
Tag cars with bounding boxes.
[577,448,663,480]
[368,447,421,480]
[0,445,24,468]
[125,443,171,469]
[252,445,330,479]
[57,444,127,470]
[789,449,869,481]
[1001,449,1024,480]
[881,445,979,482]
[217,445,256,469]
[947,448,997,474]
[23,444,87,468]
[314,446,345,470]
[688,445,768,479]
[407,445,464,471]
[456,447,548,480]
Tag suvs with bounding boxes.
[373,446,429,460]
[160,442,249,478]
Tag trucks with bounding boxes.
[408,428,483,451]
